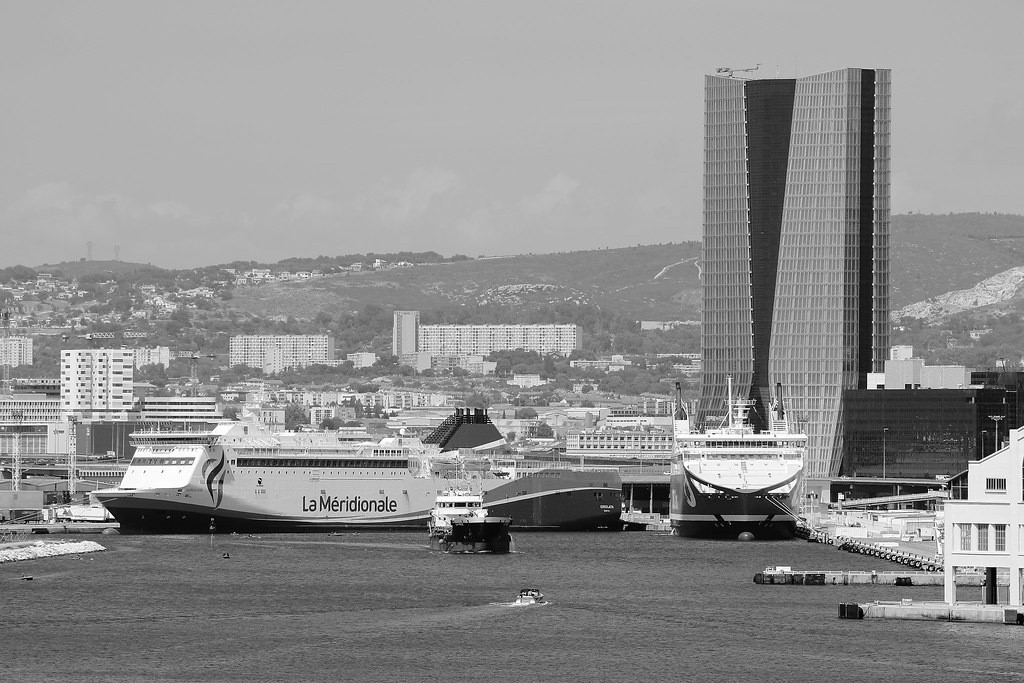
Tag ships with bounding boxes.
[88,416,436,529]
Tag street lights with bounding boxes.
[981,430,988,459]
[845,491,851,510]
[988,413,1005,452]
[882,427,889,478]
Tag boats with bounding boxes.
[484,466,636,534]
[21,575,33,580]
[423,456,515,557]
[670,374,813,539]
[222,555,230,559]
[518,589,544,608]
